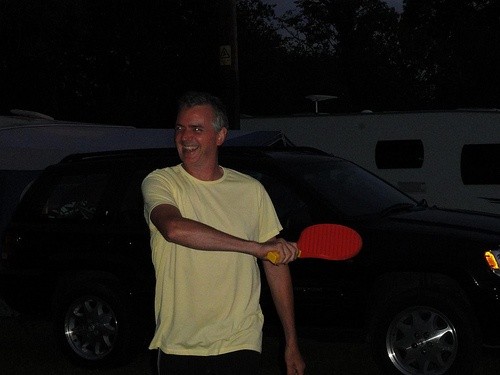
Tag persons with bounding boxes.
[140,92,306,375]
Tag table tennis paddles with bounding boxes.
[266,222,362,265]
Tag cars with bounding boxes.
[0,146,500,375]
